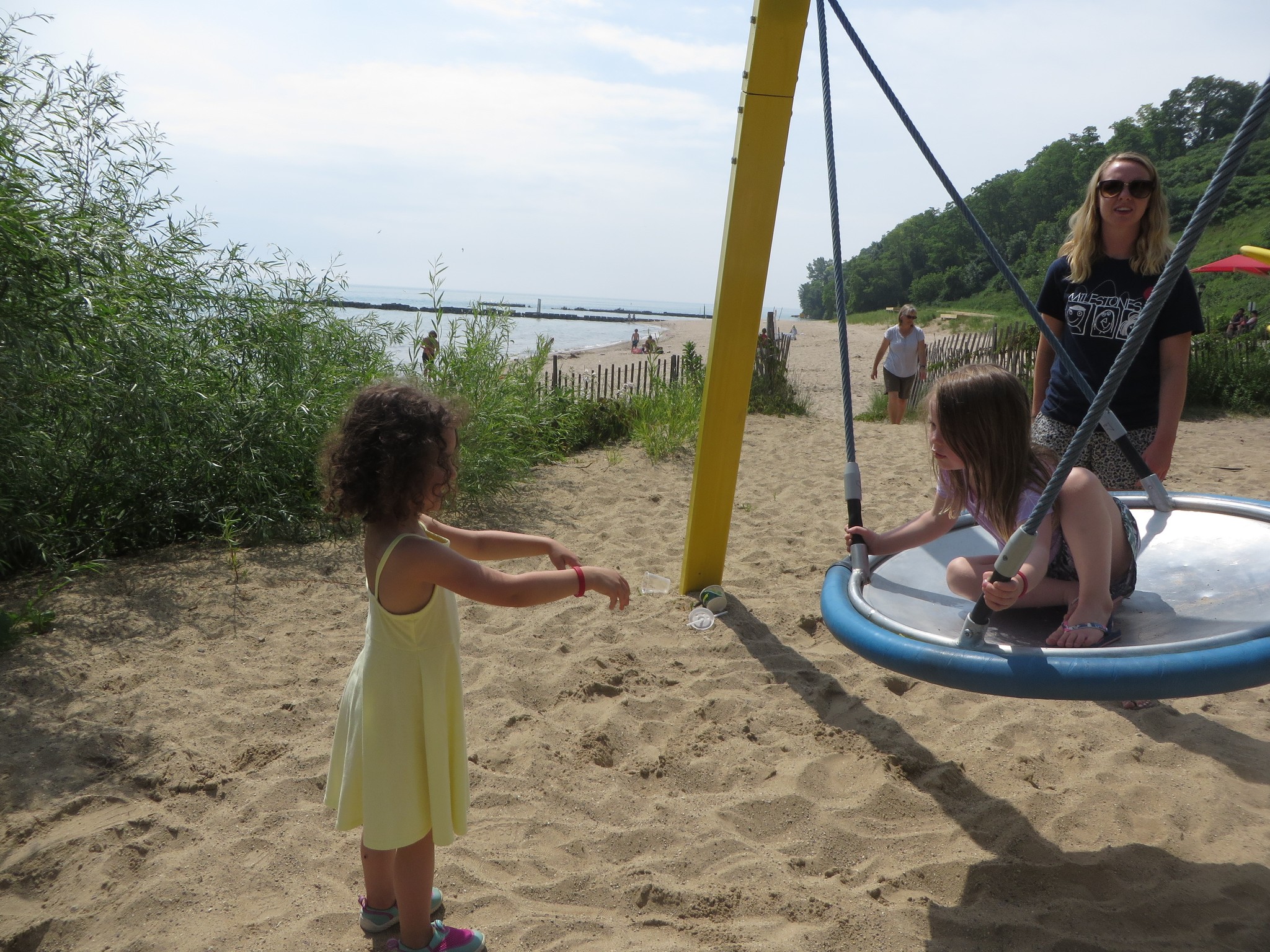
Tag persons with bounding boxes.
[1032,153,1260,710]
[845,364,1141,647]
[871,304,929,424]
[628,313,657,353]
[321,383,631,952]
[420,331,441,383]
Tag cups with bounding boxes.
[642,571,671,594]
[700,585,727,612]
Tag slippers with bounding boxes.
[1044,622,1122,648]
[1068,592,1124,614]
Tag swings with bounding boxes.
[814,0,1267,702]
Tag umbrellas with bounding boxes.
[1190,255,1270,274]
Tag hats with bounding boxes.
[792,325,795,327]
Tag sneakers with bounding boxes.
[358,887,443,933]
[385,919,485,952]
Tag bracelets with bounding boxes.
[1016,571,1027,599]
[571,565,585,599]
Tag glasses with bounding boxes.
[1096,180,1155,199]
[904,315,917,319]
